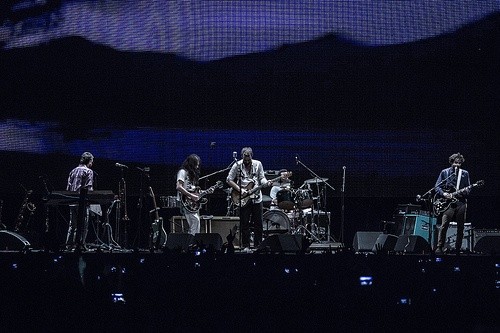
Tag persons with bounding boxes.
[335,242,385,255]
[66,152,94,252]
[270,169,290,209]
[176,153,214,236]
[226,147,274,251]
[181,228,236,252]
[435,154,470,253]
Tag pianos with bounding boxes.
[42,189,115,252]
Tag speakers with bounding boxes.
[194,233,223,255]
[472,236,500,255]
[37,234,71,252]
[261,235,299,255]
[353,232,382,251]
[105,220,146,248]
[166,233,193,250]
[372,233,399,253]
[394,235,431,254]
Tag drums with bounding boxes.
[160,195,178,208]
[276,190,294,210]
[262,209,291,241]
[295,190,314,209]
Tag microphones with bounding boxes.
[115,163,128,168]
[232,151,238,158]
[295,156,298,165]
[454,167,457,175]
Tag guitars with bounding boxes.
[149,186,167,249]
[180,180,224,214]
[432,177,490,216]
[231,171,293,208]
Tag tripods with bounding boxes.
[287,164,337,245]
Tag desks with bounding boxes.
[170,215,241,250]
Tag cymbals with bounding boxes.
[304,177,329,183]
